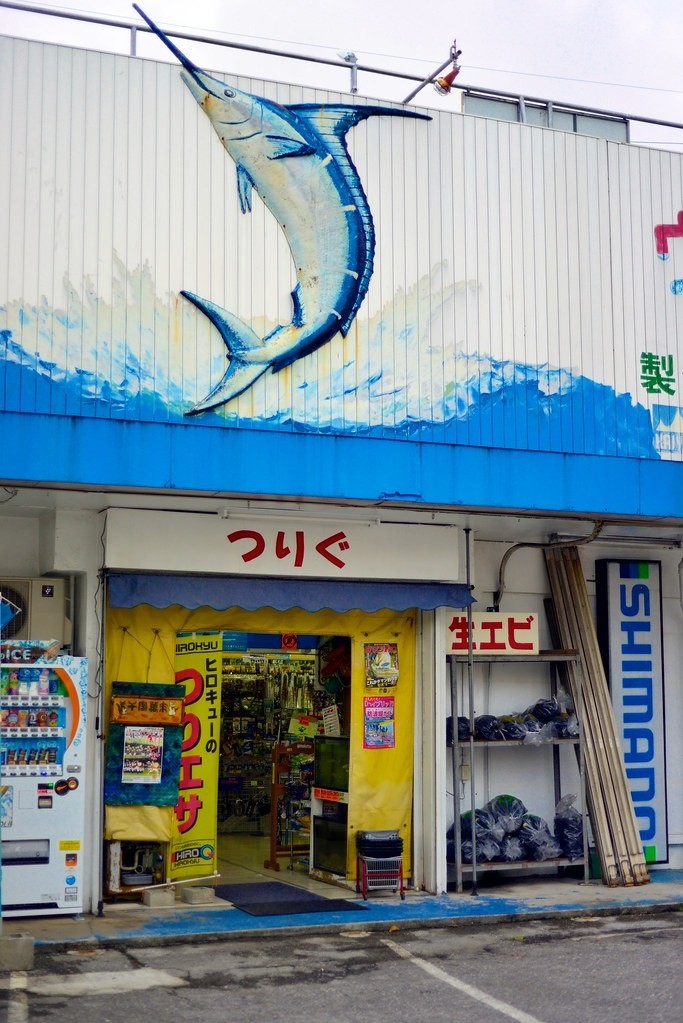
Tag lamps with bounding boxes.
[434,64,459,95]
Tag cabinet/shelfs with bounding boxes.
[442,652,591,894]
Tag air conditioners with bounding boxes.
[0,577,76,655]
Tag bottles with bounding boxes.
[49,709,58,727]
[8,712,18,727]
[38,710,48,727]
[28,711,37,726]
